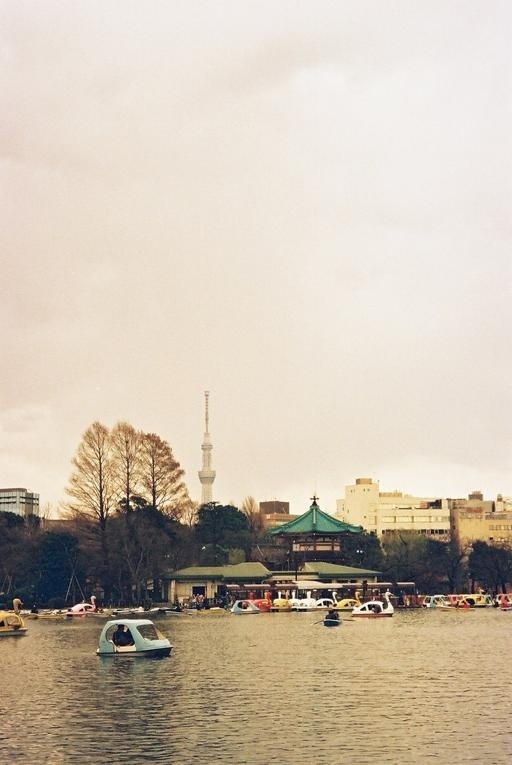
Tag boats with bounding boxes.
[352,591,396,618]
[21,605,198,617]
[322,617,342,627]
[95,618,174,657]
[404,593,512,609]
[67,595,98,616]
[0,597,28,638]
[230,589,363,614]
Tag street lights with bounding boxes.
[292,540,301,581]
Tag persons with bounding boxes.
[94,606,103,613]
[455,600,467,608]
[369,604,385,614]
[112,624,135,647]
[31,606,38,614]
[242,604,247,609]
[195,593,240,610]
[176,606,184,612]
[281,591,344,606]
[326,610,339,620]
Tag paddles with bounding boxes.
[314,620,324,624]
[340,619,356,621]
